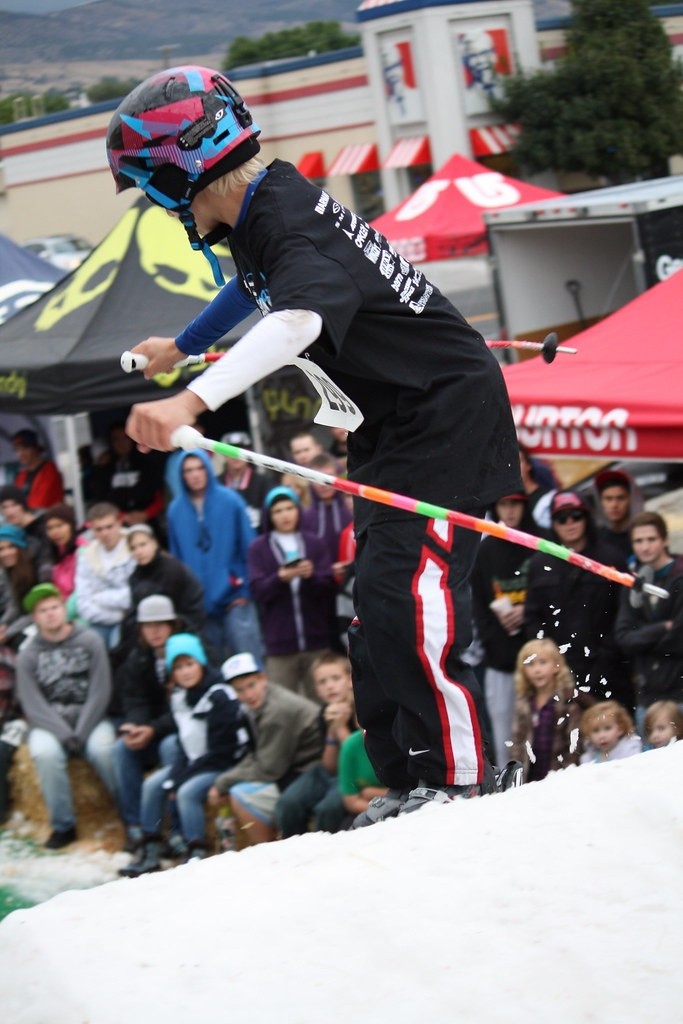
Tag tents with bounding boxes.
[0,191,264,412]
[368,154,564,265]
[501,265,683,464]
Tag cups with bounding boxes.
[491,594,520,635]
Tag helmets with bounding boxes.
[103,66,263,207]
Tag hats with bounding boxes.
[22,583,58,611]
[222,432,251,447]
[136,596,178,623]
[0,524,27,546]
[165,633,207,671]
[44,504,74,524]
[550,491,590,513]
[265,485,299,507]
[219,652,260,682]
[1,484,23,502]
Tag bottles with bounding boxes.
[215,807,237,853]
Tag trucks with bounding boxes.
[478,173,683,366]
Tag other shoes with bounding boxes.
[401,786,476,815]
[118,856,160,877]
[44,828,77,848]
[125,839,143,852]
[345,792,406,830]
[166,843,187,861]
[191,847,209,861]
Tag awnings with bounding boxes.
[381,136,432,170]
[296,152,323,179]
[327,144,380,178]
[470,122,525,156]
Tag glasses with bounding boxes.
[554,514,583,524]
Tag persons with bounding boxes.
[0,419,388,879]
[104,65,526,829]
[466,442,683,784]
